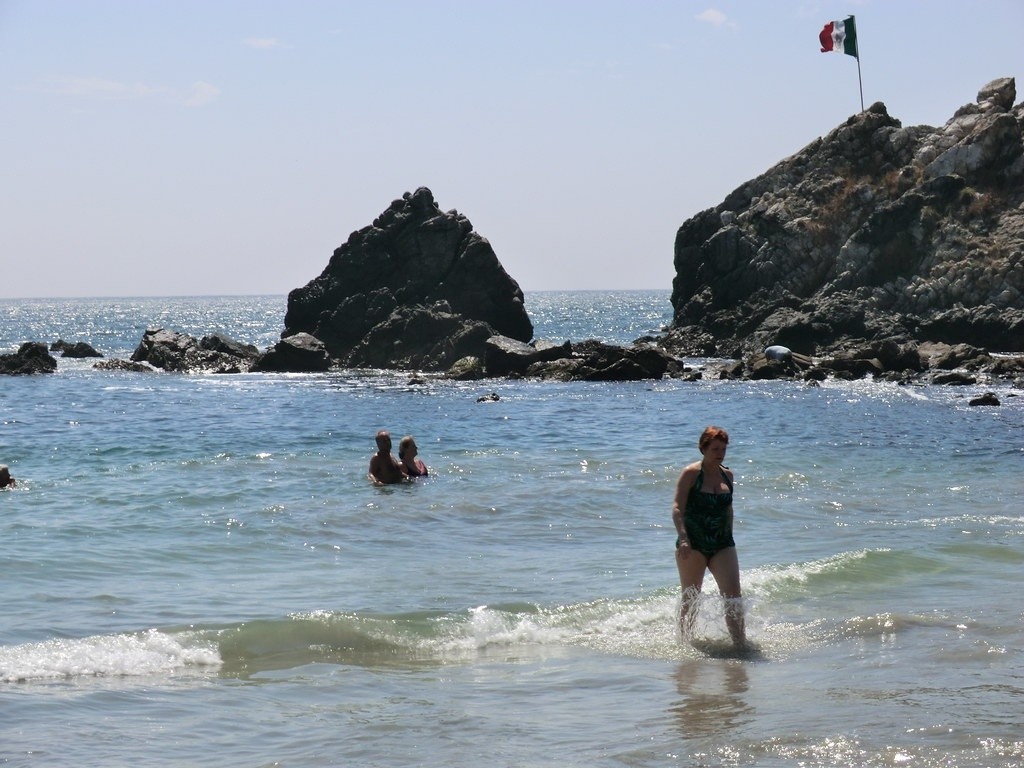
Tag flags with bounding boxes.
[819,17,857,58]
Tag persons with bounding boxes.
[368,431,428,486]
[0,464,15,488]
[672,425,745,643]
